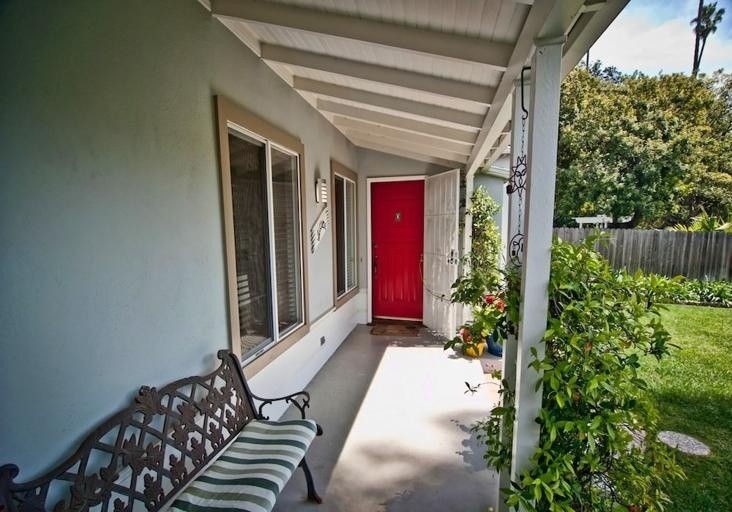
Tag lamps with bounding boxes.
[315,178,327,207]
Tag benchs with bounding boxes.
[1,349,324,511]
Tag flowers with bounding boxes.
[451,271,507,345]
[443,318,489,356]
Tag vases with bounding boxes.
[485,334,502,357]
[462,343,483,357]
